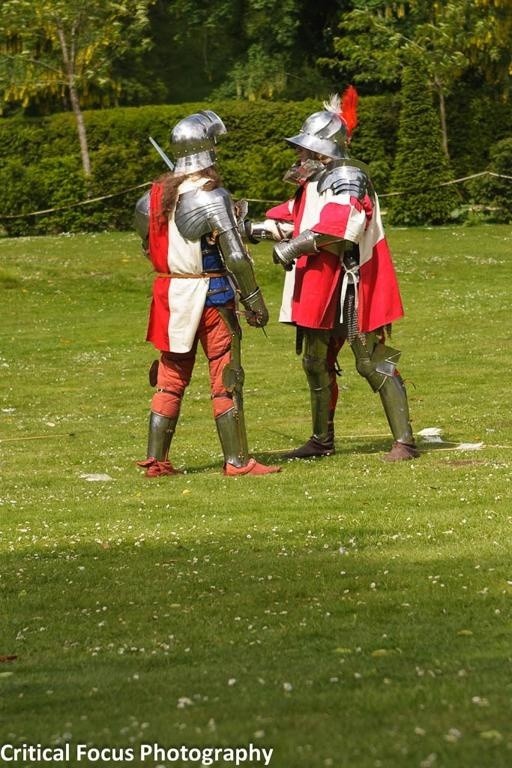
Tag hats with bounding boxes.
[281,110,353,162]
[169,108,228,179]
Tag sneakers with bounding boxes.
[223,458,282,477]
[280,436,338,459]
[135,457,176,478]
[382,441,421,463]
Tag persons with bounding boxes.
[133,110,283,479]
[242,86,422,463]
